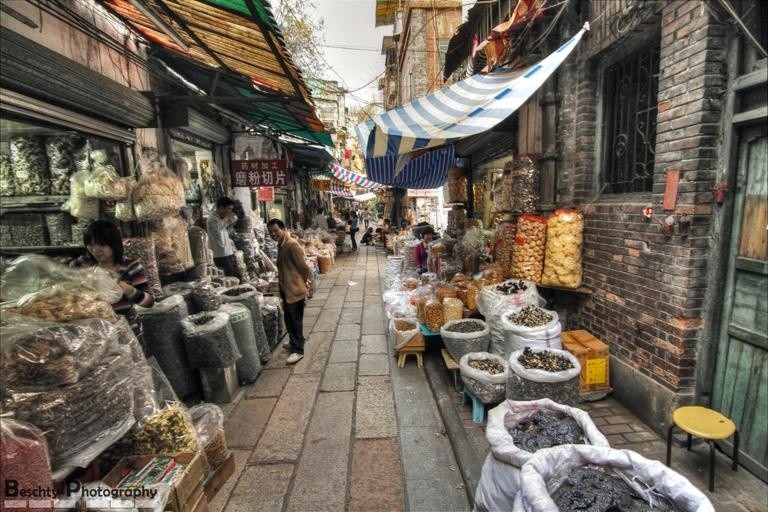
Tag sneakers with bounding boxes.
[287,353,304,363]
[283,342,291,348]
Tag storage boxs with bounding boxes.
[102,452,205,509]
[561,330,610,393]
[390,324,425,347]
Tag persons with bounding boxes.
[69,218,155,350]
[266,217,314,365]
[413,225,434,272]
[205,195,239,276]
[311,205,392,252]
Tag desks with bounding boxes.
[421,322,442,335]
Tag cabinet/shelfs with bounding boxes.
[491,211,593,294]
[0,195,123,256]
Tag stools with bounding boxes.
[440,348,462,394]
[398,351,424,369]
[463,386,484,424]
[667,405,739,491]
